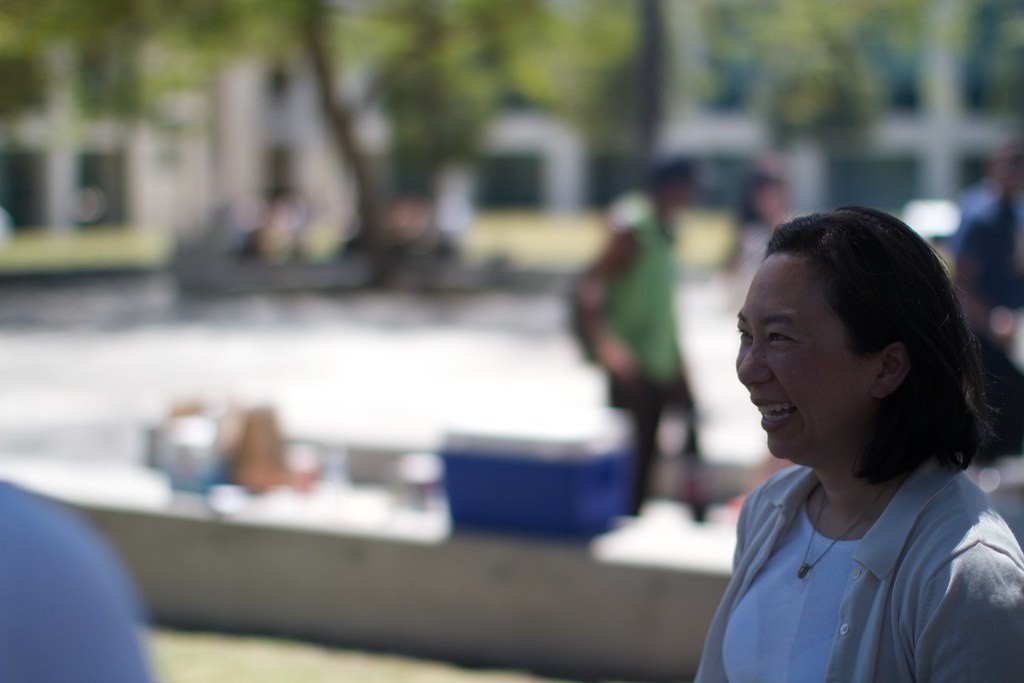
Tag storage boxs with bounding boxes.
[436,403,638,544]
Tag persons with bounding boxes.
[168,137,1023,522]
[690,201,1024,683]
